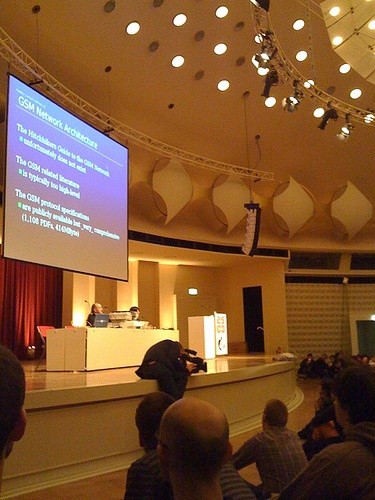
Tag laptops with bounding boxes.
[93,315,110,327]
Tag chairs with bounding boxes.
[34,326,55,371]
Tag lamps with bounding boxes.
[340,122,355,137]
[255,52,270,76]
[317,108,339,131]
[261,69,279,98]
[286,94,302,106]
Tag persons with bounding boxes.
[126,340,375,500]
[87,303,103,326]
[0,343,27,483]
[130,306,140,320]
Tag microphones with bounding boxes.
[142,316,154,329]
[185,349,197,356]
[85,301,90,309]
[105,306,122,328]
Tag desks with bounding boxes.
[46,327,180,372]
